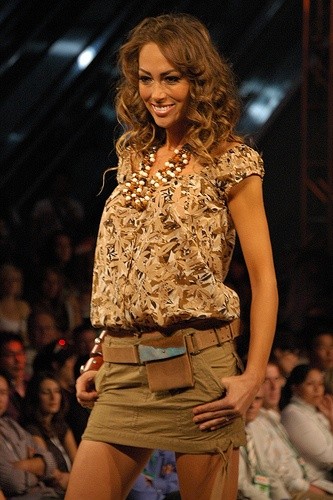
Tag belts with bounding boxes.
[104,317,244,364]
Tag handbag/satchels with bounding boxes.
[137,333,196,393]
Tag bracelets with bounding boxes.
[80,330,110,373]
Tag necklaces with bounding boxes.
[119,131,201,212]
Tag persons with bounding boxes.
[128,449,183,500]
[61,13,283,500]
[0,331,40,428]
[262,362,333,499]
[238,386,293,499]
[0,207,98,387]
[226,256,333,397]
[61,356,93,465]
[283,365,333,494]
[0,371,66,499]
[20,373,74,496]
